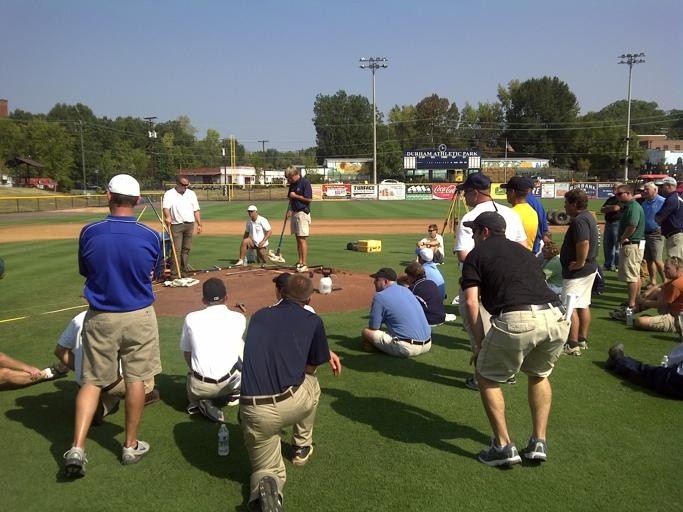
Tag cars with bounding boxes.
[69,180,102,190]
[380,179,404,184]
[229,182,244,189]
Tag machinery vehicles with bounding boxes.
[546,181,606,225]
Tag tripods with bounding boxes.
[441,194,469,236]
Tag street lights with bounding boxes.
[358,57,388,184]
[615,53,645,184]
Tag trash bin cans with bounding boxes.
[154,231,171,280]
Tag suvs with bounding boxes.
[612,174,669,195]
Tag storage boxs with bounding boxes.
[358,240,382,252]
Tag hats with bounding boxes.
[463,211,506,231]
[456,173,490,190]
[272,273,292,288]
[656,177,677,186]
[203,278,226,301]
[369,268,397,281]
[416,248,433,262]
[500,176,529,191]
[248,205,257,211]
[108,174,140,197]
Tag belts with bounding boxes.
[666,231,681,238]
[622,241,639,245]
[400,338,430,345]
[194,363,237,384]
[241,386,299,405]
[503,301,561,312]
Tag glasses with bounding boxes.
[428,229,435,232]
[179,181,189,186]
[617,192,628,196]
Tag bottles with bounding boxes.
[626,306,633,326]
[661,355,669,368]
[217,424,230,456]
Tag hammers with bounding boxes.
[236,303,247,312]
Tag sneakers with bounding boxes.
[199,398,224,422]
[289,262,308,273]
[291,445,313,465]
[465,377,481,390]
[235,257,247,266]
[562,339,589,356]
[521,436,548,462]
[225,394,240,406]
[121,440,150,465]
[145,390,160,405]
[507,377,516,385]
[362,341,381,352]
[609,284,658,321]
[478,438,522,466]
[41,365,69,381]
[600,264,618,272]
[607,343,625,370]
[258,476,284,512]
[63,447,88,479]
[171,272,187,280]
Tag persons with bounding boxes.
[609,342,683,399]
[600,176,683,336]
[460,211,571,465]
[285,165,312,273]
[268,273,316,315]
[162,177,202,281]
[238,275,341,512]
[452,172,552,391]
[235,205,272,266]
[0,352,69,386]
[559,188,599,357]
[63,174,162,477]
[362,224,446,359]
[54,311,160,425]
[181,278,246,422]
